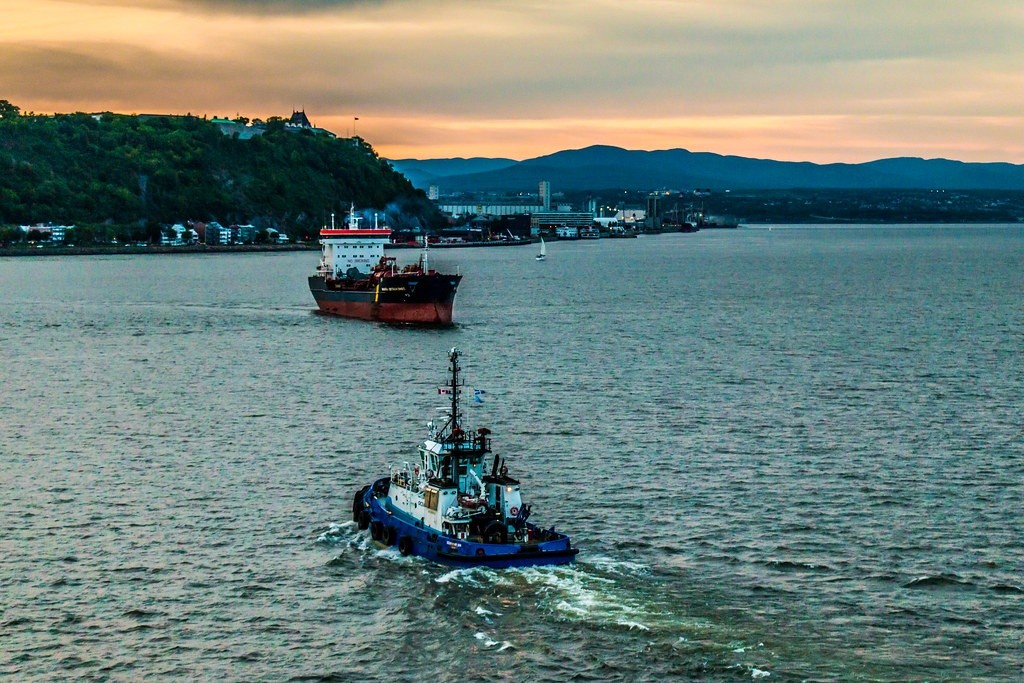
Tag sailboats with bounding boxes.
[536,238,548,261]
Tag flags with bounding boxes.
[438,389,450,394]
[474,390,486,404]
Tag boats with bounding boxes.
[352,347,579,569]
[308,201,465,330]
[580,226,600,239]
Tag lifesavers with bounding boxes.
[424,468,434,479]
[510,506,519,516]
[499,465,508,475]
